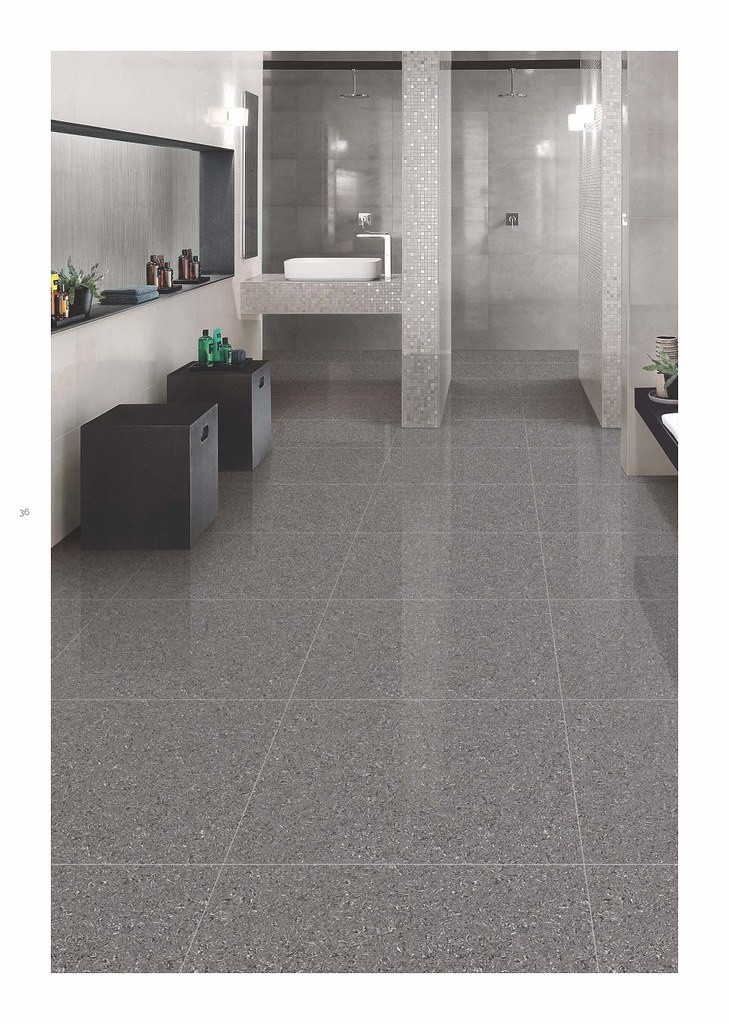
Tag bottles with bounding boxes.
[146,255,159,285]
[219,337,232,368]
[197,330,213,366]
[178,249,190,280]
[160,261,173,290]
[191,255,201,280]
[54,284,69,320]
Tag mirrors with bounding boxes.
[243,91,258,258]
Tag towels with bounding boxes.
[98,285,159,304]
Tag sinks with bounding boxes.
[283,257,383,282]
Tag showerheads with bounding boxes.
[497,93,527,100]
[338,93,370,100]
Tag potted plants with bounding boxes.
[643,346,678,399]
[51,255,106,317]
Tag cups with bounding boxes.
[232,349,246,365]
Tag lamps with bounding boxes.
[230,108,249,126]
[209,108,230,125]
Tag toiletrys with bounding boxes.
[178,249,202,280]
[146,254,173,289]
[51,270,69,320]
[197,327,233,368]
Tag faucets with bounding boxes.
[356,232,392,279]
